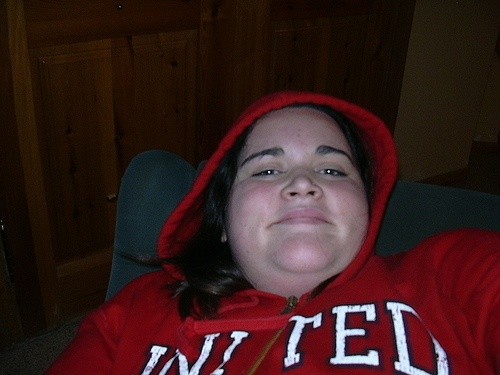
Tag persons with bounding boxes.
[44,89,500,375]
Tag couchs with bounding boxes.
[0,147,500,375]
